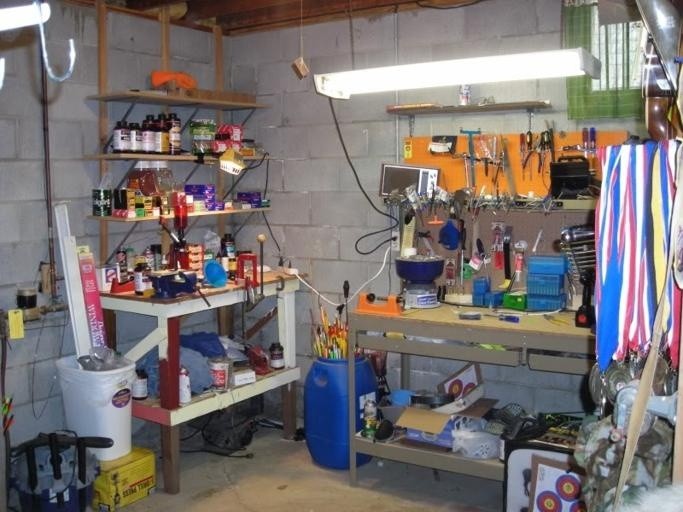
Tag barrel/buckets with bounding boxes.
[301,349,380,470]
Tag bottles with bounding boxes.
[111,120,130,153]
[141,114,156,154]
[220,232,235,260]
[153,114,170,156]
[133,263,152,296]
[269,342,284,369]
[130,368,147,401]
[166,113,181,154]
[128,122,142,152]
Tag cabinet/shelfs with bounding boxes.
[84,1,307,495]
[343,291,627,488]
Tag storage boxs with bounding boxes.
[84,447,158,511]
[394,386,500,451]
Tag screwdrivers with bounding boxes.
[476,238,491,286]
[590,127,595,169]
[527,131,532,181]
[520,133,525,182]
[482,313,520,322]
[460,227,466,293]
[582,128,588,160]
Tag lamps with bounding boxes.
[1,1,52,32]
[311,47,603,99]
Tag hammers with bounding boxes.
[491,222,513,280]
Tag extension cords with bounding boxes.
[277,265,298,275]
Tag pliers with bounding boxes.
[523,142,542,173]
[543,314,569,325]
